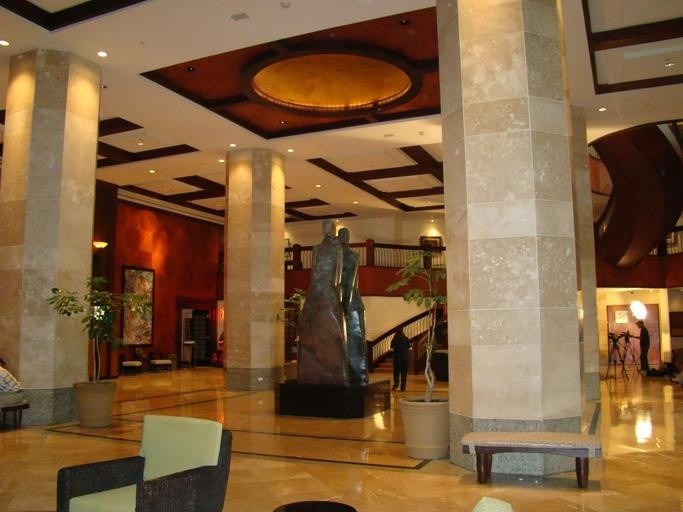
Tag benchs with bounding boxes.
[462,429,602,488]
[148,353,173,373]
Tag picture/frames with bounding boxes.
[419,235,442,256]
[120,265,153,347]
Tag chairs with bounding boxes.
[117,353,144,374]
[56,412,234,511]
[0,361,29,429]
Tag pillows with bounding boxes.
[0,367,23,394]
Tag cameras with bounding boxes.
[608,333,622,350]
[621,331,634,342]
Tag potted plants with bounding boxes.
[42,276,156,428]
[384,248,450,463]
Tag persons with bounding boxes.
[337,228,368,384]
[297,220,348,385]
[0,366,23,408]
[628,321,649,372]
[670,370,683,384]
[389,326,412,391]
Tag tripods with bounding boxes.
[606,348,629,381]
[622,343,640,376]
